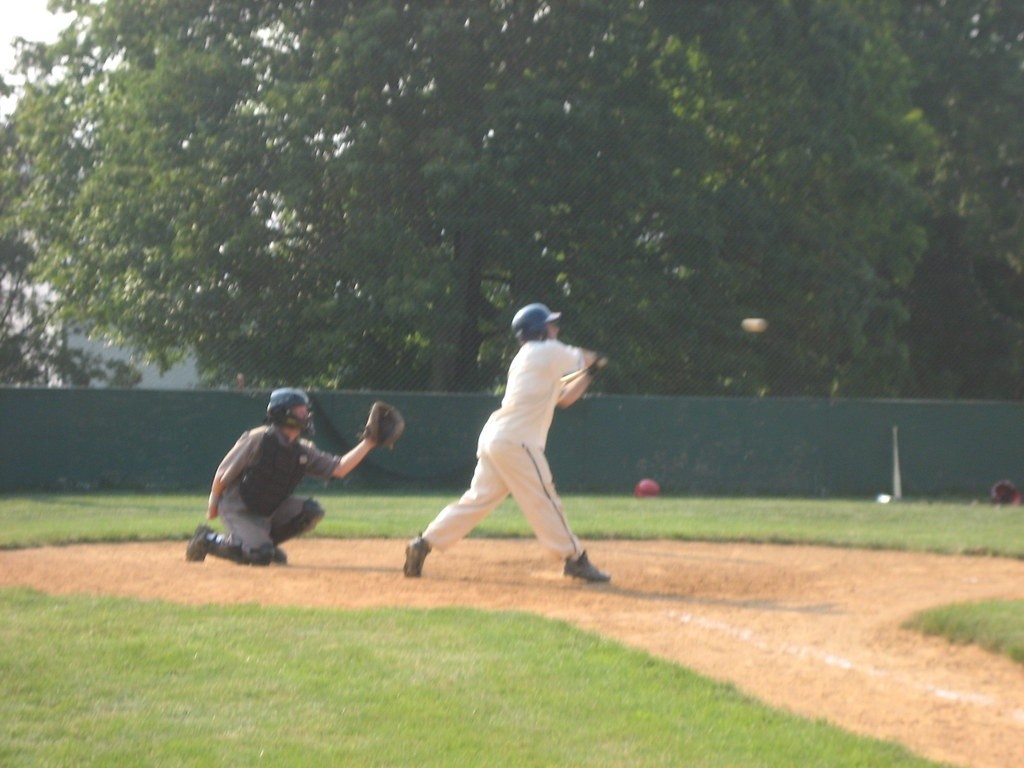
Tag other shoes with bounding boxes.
[187,524,213,561]
[270,547,287,563]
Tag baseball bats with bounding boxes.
[892,424,901,498]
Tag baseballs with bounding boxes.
[741,316,769,334]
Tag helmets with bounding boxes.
[267,388,308,417]
[510,303,561,340]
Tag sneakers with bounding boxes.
[403,532,431,577]
[564,550,612,582]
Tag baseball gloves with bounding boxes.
[361,401,405,449]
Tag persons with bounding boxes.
[403,304,611,582]
[186,388,403,567]
